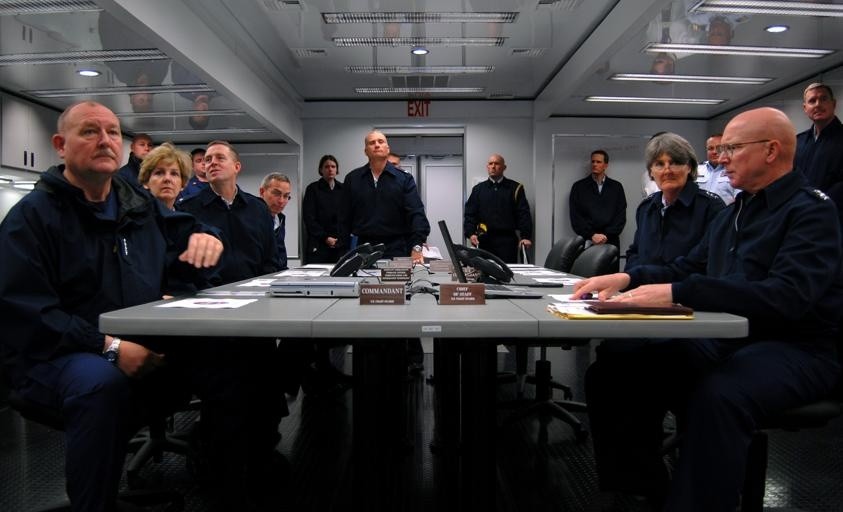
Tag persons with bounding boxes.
[622,1,689,83]
[343,130,430,371]
[463,154,532,263]
[98,7,170,112]
[170,62,220,130]
[2,100,230,512]
[570,150,627,273]
[302,155,344,265]
[574,107,842,512]
[387,153,432,251]
[625,132,726,444]
[119,134,292,301]
[692,5,752,59]
[794,83,842,205]
[638,131,742,205]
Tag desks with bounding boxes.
[99,264,750,512]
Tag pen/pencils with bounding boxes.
[193,301,229,305]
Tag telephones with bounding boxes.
[360,243,385,269]
[456,246,514,284]
[330,243,373,277]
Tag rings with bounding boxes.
[628,293,633,299]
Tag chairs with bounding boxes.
[497,234,584,398]
[740,399,843,512]
[515,243,618,446]
[126,411,192,489]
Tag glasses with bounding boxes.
[717,140,771,158]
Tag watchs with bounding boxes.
[412,245,422,251]
[104,336,122,363]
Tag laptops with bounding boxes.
[438,219,543,299]
[269,277,365,298]
